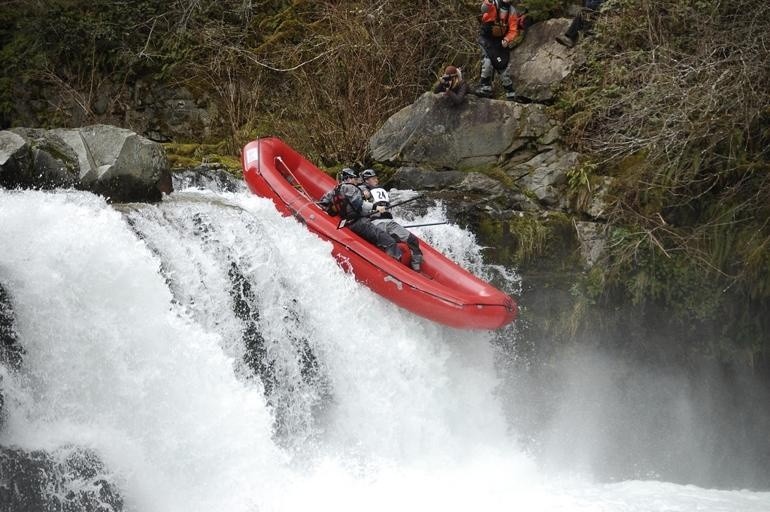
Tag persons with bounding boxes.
[555,16,582,47]
[318,168,402,260]
[432,65,466,103]
[358,170,423,272]
[478,0,520,100]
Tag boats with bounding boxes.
[243,138,518,330]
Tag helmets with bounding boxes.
[361,169,376,181]
[337,168,360,178]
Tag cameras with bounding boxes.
[441,76,453,87]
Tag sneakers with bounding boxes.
[475,89,495,98]
[555,36,574,48]
[418,271,433,280]
[507,96,518,101]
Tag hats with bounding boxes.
[445,66,457,75]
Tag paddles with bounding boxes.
[336,193,423,229]
